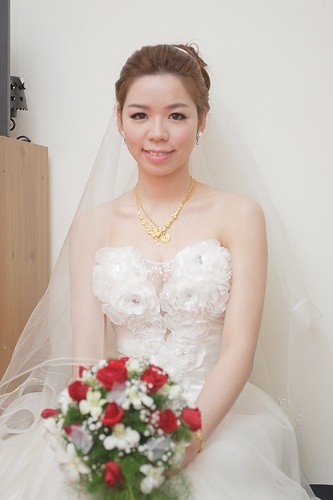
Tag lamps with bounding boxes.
[9,75,30,117]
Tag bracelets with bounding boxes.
[195,430,205,453]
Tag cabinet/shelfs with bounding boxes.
[1,134,49,412]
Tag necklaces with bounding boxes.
[134,178,200,248]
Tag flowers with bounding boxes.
[45,356,201,499]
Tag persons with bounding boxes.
[0,39,301,494]
